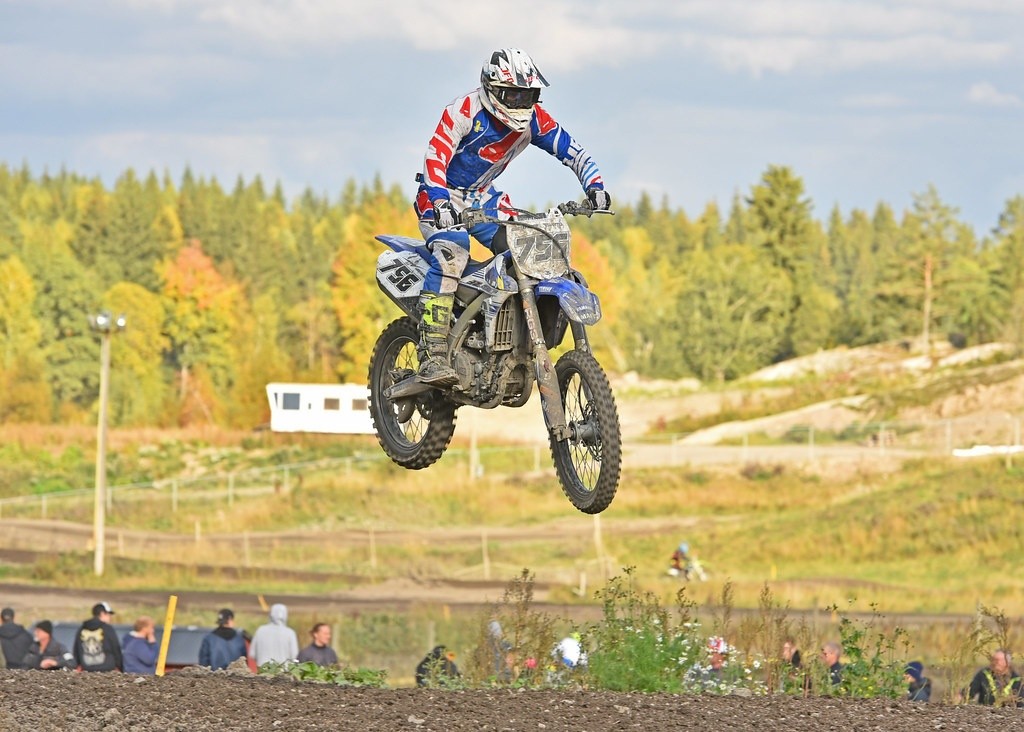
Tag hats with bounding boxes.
[704,636,729,655]
[903,661,924,681]
[2,608,14,623]
[92,603,114,617]
[37,620,52,634]
[215,610,233,626]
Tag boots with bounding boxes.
[419,289,460,386]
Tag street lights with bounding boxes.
[87,312,128,574]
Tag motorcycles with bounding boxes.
[366,199,622,513]
[662,560,713,582]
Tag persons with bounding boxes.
[414,47,610,385]
[670,544,694,580]
[954,651,1024,708]
[0,602,340,673]
[416,622,586,686]
[685,638,934,700]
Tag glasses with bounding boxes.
[501,89,535,109]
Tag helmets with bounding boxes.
[480,46,550,133]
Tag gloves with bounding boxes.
[588,189,611,210]
[433,200,460,230]
